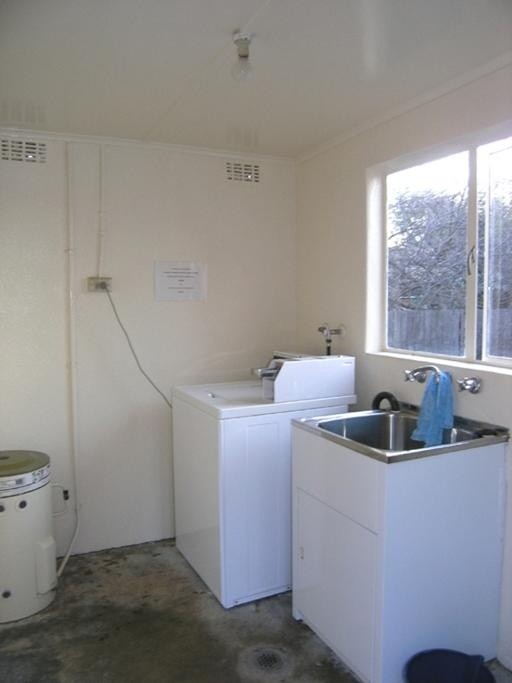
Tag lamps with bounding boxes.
[229,32,254,83]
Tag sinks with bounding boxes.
[292,409,509,460]
[208,385,271,401]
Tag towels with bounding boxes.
[410,372,455,447]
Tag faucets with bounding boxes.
[266,364,267,366]
[404,365,451,392]
[251,366,279,379]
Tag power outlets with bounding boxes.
[88,277,113,293]
